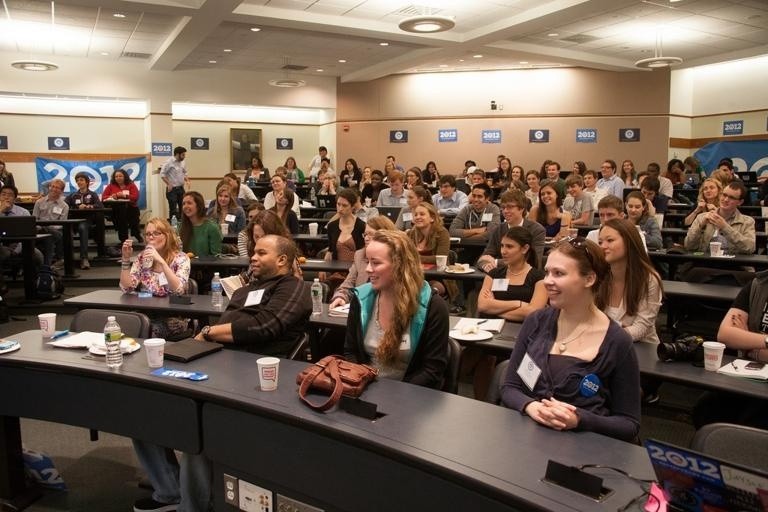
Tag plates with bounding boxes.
[88,344,140,356]
[0,342,21,355]
[449,329,494,343]
[445,267,475,274]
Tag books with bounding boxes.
[44,330,104,349]
[714,357,768,383]
[163,337,224,363]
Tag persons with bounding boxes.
[159,146,192,222]
[0,186,44,288]
[118,217,191,299]
[63,172,105,269]
[101,168,144,243]
[0,160,15,189]
[341,228,449,393]
[717,269,768,365]
[30,178,71,250]
[131,234,314,511]
[497,236,642,443]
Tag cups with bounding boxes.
[143,338,165,369]
[170,174,372,236]
[256,356,280,392]
[565,228,578,242]
[37,312,56,338]
[702,342,726,372]
[435,255,448,272]
[708,241,721,256]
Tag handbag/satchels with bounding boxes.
[296,352,376,410]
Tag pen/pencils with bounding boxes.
[477,320,487,324]
[50,330,69,339]
[731,360,739,371]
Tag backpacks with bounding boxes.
[36,266,63,301]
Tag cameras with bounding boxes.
[657,333,703,364]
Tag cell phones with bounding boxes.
[132,241,145,252]
[745,361,764,371]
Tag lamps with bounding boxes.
[8,38,61,74]
[396,6,458,35]
[266,51,308,88]
[632,27,683,70]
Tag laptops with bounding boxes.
[686,173,700,186]
[316,194,337,208]
[376,206,402,225]
[735,171,757,183]
[0,215,36,236]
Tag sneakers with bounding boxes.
[79,258,90,269]
[129,496,183,512]
[135,233,144,242]
[53,260,65,269]
[448,304,468,317]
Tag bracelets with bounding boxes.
[119,259,131,270]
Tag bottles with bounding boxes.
[210,272,223,307]
[104,316,123,367]
[310,279,322,314]
[140,241,155,269]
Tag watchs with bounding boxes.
[764,335,768,348]
[201,325,215,342]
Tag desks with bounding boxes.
[0,164,767,511]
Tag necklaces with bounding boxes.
[557,308,593,352]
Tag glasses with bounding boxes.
[560,236,595,266]
[144,231,162,237]
